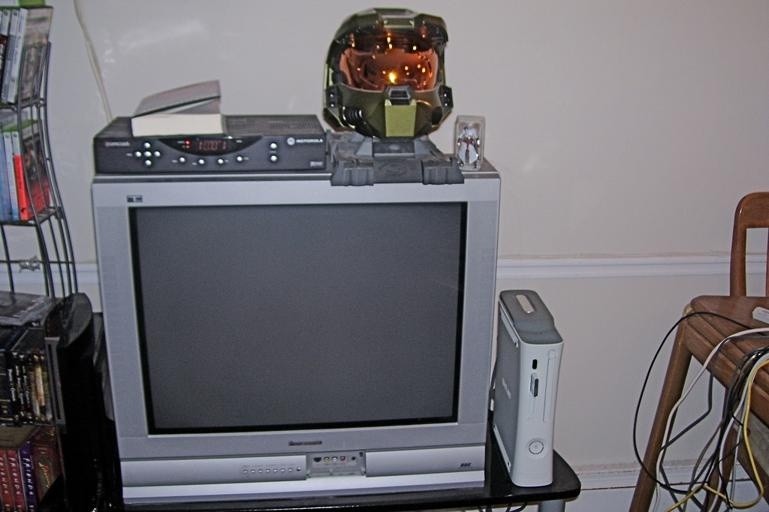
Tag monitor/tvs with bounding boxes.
[129,79,227,139]
[0,0,53,225]
[0,288,68,512]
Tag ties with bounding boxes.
[91,152,501,503]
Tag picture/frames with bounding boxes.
[38,411,582,512]
[629,295,765,512]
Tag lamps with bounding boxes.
[702,190,765,511]
